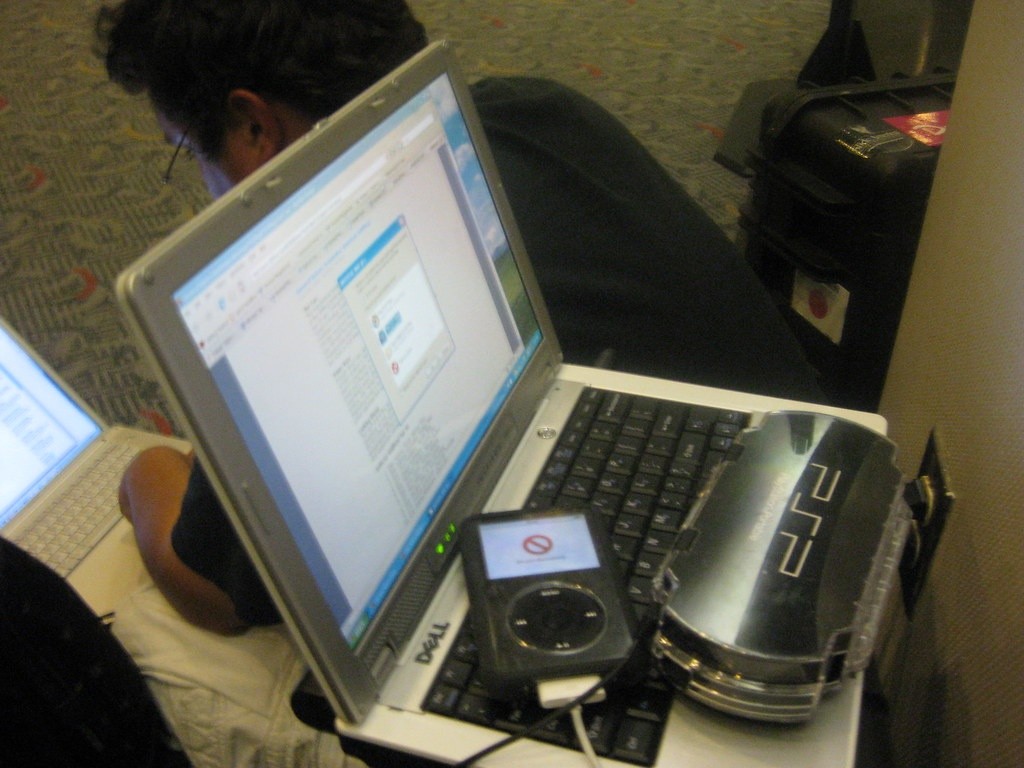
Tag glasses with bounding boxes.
[159,122,195,193]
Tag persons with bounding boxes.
[92,0,896,768]
[0,537,192,768]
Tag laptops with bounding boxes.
[0,318,193,627]
[117,40,889,768]
